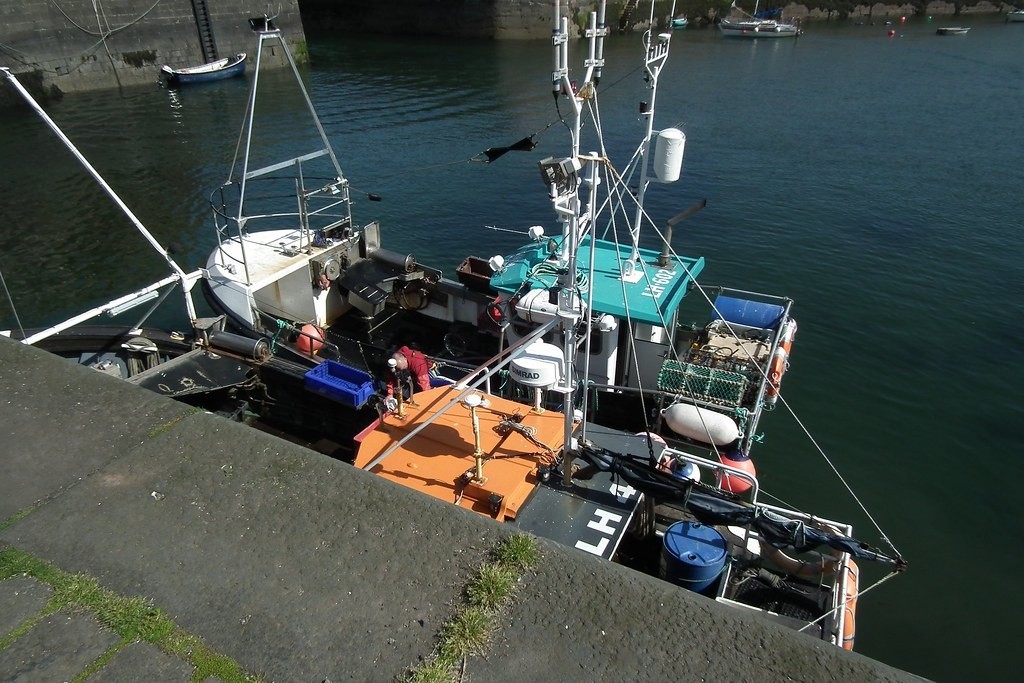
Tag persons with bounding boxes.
[383,346,452,405]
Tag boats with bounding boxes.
[1005,11,1024,22]
[936,26,972,35]
[716,1,804,37]
[200,0,798,489]
[160,52,247,87]
[668,18,687,26]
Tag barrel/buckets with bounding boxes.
[660,520,728,593]
